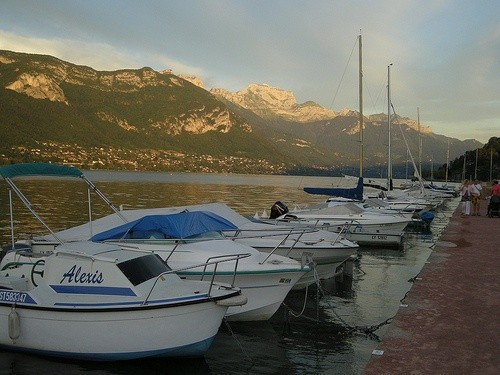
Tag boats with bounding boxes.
[30,200,361,292]
[246,201,420,245]
[1,161,320,323]
[0,240,253,363]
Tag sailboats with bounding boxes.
[297,27,500,231]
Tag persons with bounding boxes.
[485,178,500,216]
[461,179,483,216]
